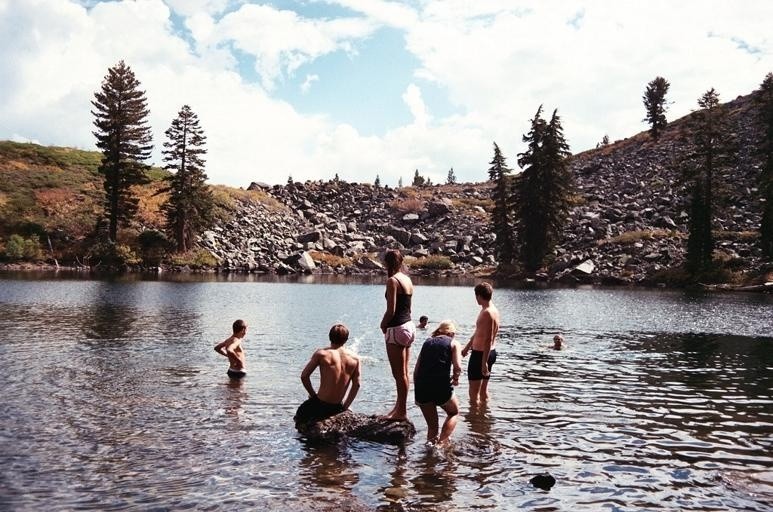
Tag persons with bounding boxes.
[547,335,565,351]
[214,319,247,380]
[379,249,415,421]
[413,319,461,444]
[461,280,499,402]
[415,315,428,328]
[293,325,360,429]
